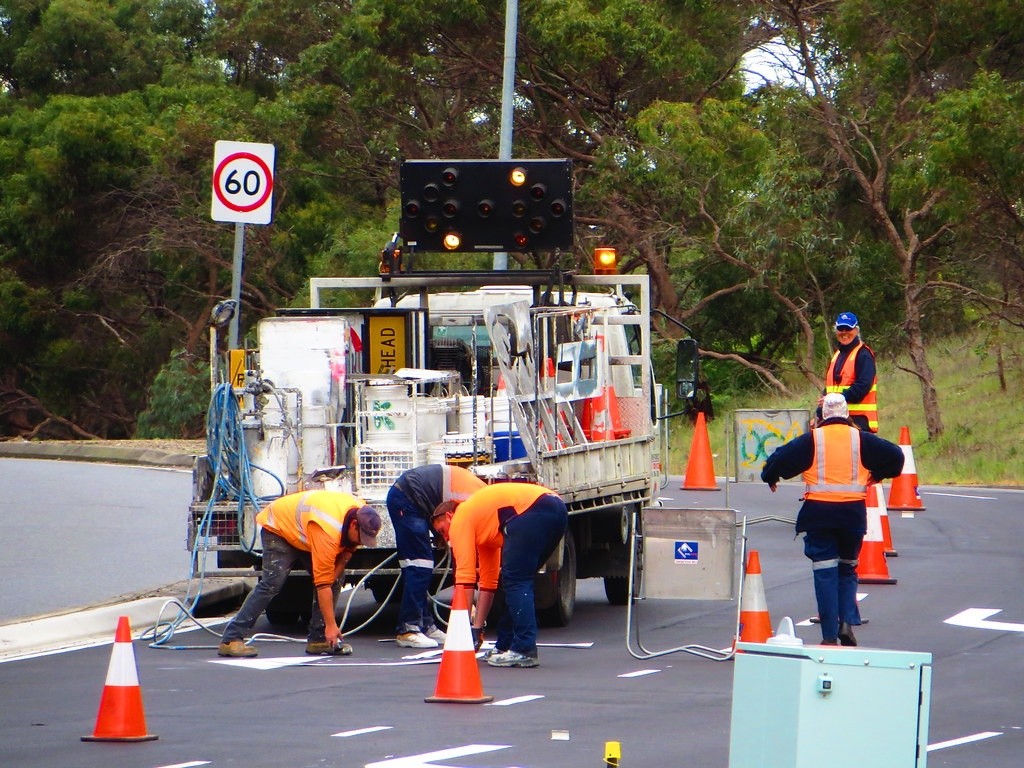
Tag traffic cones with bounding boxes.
[886,426,927,511]
[870,480,898,559]
[81,615,160,742]
[578,335,633,441]
[725,550,775,660]
[679,412,723,491]
[853,485,898,585]
[423,584,494,705]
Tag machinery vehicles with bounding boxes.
[186,156,701,629]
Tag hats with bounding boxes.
[357,505,382,547]
[835,313,859,329]
[823,393,850,419]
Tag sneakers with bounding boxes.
[305,641,353,654]
[429,628,446,645]
[218,640,257,656]
[488,649,539,667]
[397,631,439,648]
[484,646,504,661]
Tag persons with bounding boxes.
[761,393,906,646]
[216,490,380,654]
[430,483,569,668]
[385,463,487,647]
[810,312,878,433]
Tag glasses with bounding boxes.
[430,513,446,522]
[837,326,852,331]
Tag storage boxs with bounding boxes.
[351,432,526,500]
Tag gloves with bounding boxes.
[471,624,484,650]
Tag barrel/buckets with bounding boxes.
[364,385,526,463]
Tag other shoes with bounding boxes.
[838,621,857,646]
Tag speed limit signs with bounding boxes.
[210,139,275,225]
